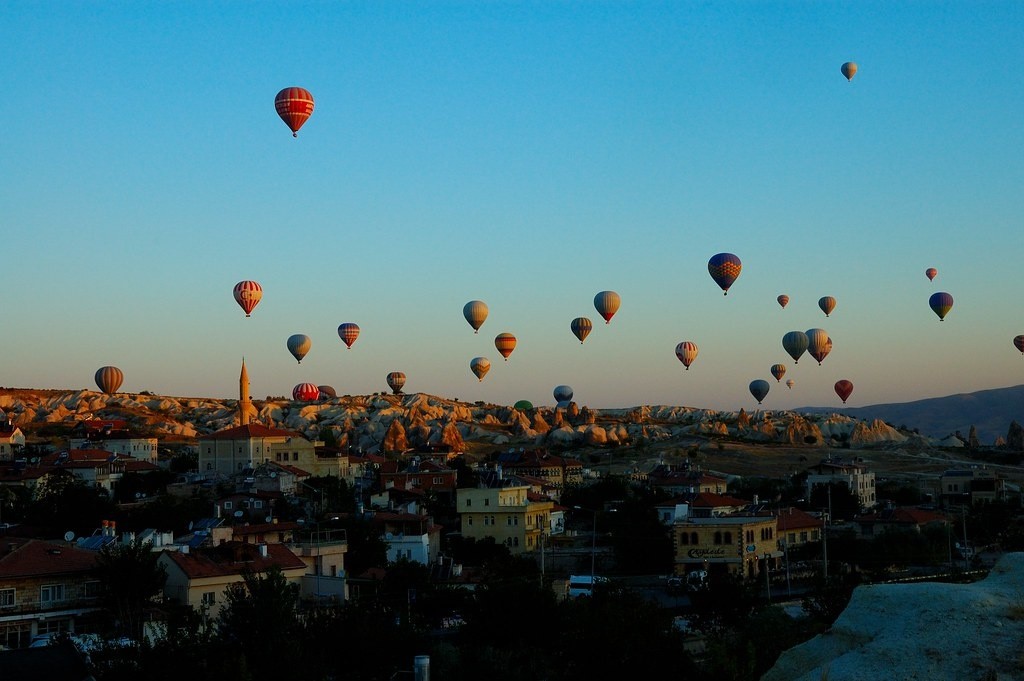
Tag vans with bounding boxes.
[960,548,973,560]
[569,575,611,600]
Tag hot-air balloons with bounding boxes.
[782,331,809,364]
[841,62,857,82]
[805,329,828,355]
[293,383,320,403]
[317,386,336,399]
[514,400,532,412]
[771,364,786,382]
[495,333,517,362]
[275,87,314,138]
[786,380,794,389]
[749,380,770,404]
[387,372,406,395]
[287,334,311,364]
[778,295,789,309]
[471,357,490,381]
[463,300,488,334]
[594,291,621,324]
[834,380,854,403]
[819,296,836,317]
[234,281,263,318]
[929,292,954,322]
[809,337,832,366]
[338,322,359,350]
[554,385,573,402]
[926,268,937,281]
[1014,335,1024,355]
[711,253,741,297]
[571,317,592,345]
[94,366,123,393]
[675,341,699,370]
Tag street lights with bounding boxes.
[296,517,341,604]
[574,506,618,600]
[292,480,324,515]
[761,499,805,598]
[925,492,969,571]
